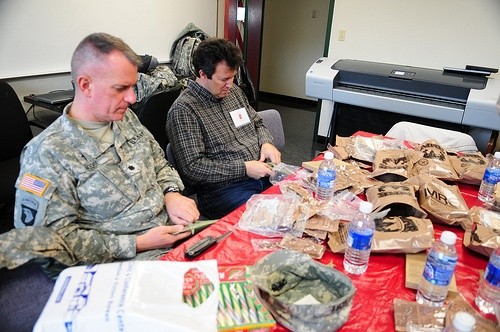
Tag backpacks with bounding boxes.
[172,36,201,78]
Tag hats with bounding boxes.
[250,248,357,332]
[0,226,79,269]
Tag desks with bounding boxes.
[159,130,500,332]
[23,91,74,130]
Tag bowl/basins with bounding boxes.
[247,249,354,332]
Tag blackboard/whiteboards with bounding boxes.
[0,0,218,80]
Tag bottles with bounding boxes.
[478,151,500,204]
[414,231,458,308]
[314,152,336,203]
[439,312,476,332]
[343,202,376,274]
[473,248,500,315]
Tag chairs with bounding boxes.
[135,87,184,160]
[0,80,56,332]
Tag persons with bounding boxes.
[14,33,201,265]
[167,39,282,219]
